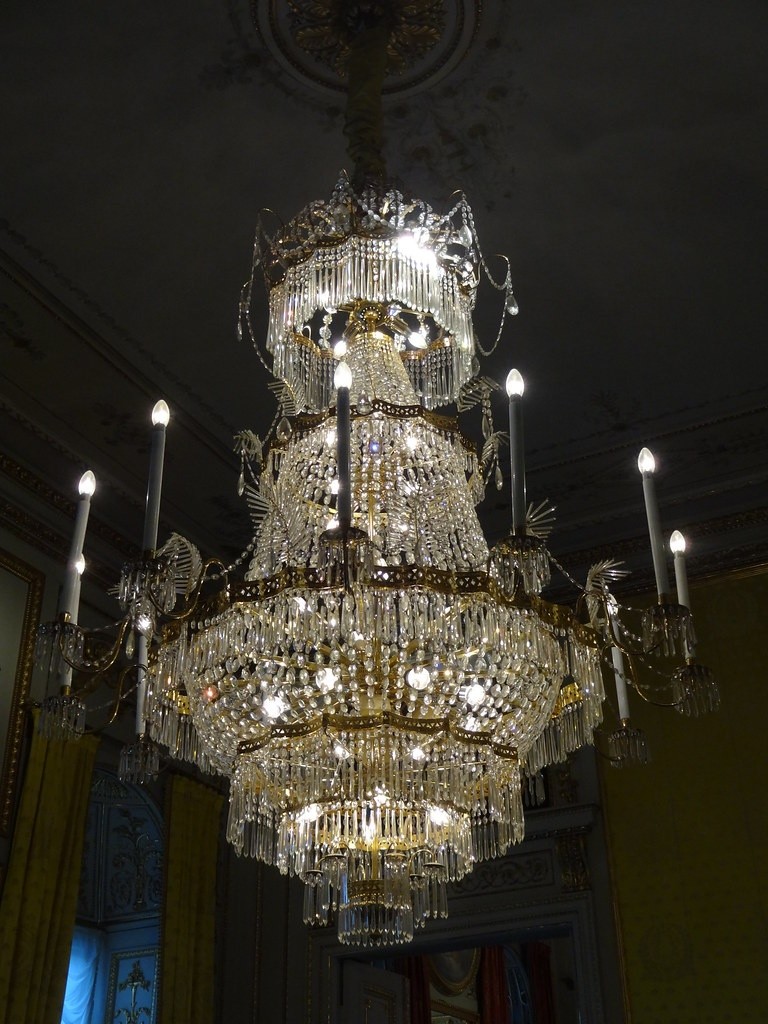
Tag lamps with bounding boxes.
[30,0,723,945]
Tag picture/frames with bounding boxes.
[429,945,482,997]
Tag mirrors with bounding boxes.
[304,891,605,1024]
